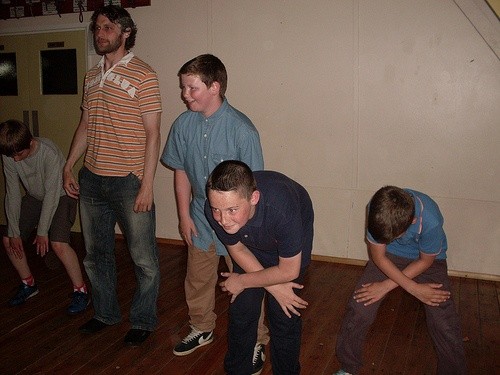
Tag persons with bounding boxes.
[0,118,90,314]
[335,185,473,375]
[203,159,315,375]
[62,4,161,347]
[159,53,267,375]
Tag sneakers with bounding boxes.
[333,369,353,375]
[9,281,39,305]
[252,343,266,375]
[67,286,90,314]
[173,328,213,356]
[124,329,151,343]
[79,318,108,332]
